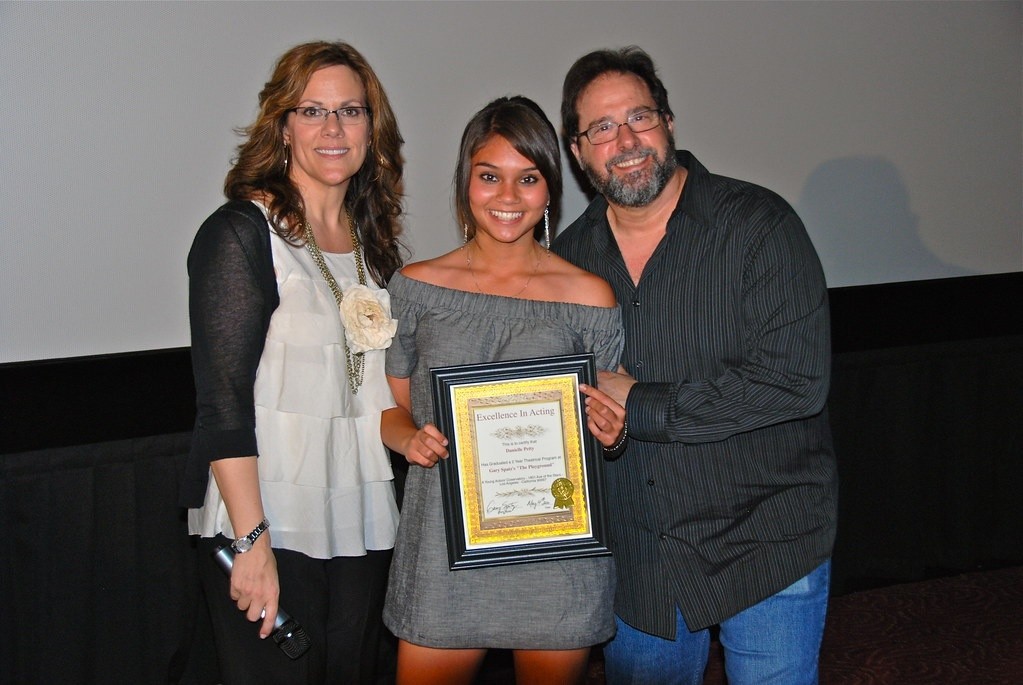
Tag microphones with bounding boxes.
[212,544,310,659]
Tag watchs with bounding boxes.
[229,518,269,555]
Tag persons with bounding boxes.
[379,95,625,685]
[183,39,402,685]
[547,43,840,685]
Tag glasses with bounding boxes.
[287,106,371,125]
[575,107,665,146]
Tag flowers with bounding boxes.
[340,282,400,357]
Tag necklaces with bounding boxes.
[462,242,543,297]
[294,200,367,396]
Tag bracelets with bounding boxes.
[603,421,627,452]
